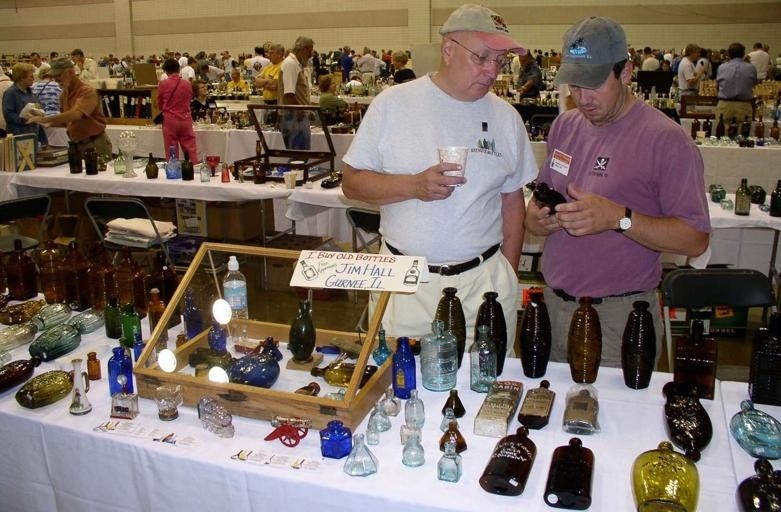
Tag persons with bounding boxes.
[342,45,415,83]
[713,42,757,122]
[50,51,58,60]
[3,63,42,137]
[533,48,537,59]
[626,44,710,99]
[198,48,256,99]
[44,59,114,161]
[160,48,196,61]
[744,43,771,82]
[313,49,341,122]
[31,52,51,74]
[516,47,541,136]
[157,58,197,166]
[254,43,286,127]
[523,16,711,373]
[342,4,540,360]
[100,50,157,76]
[544,51,549,67]
[0,67,15,140]
[278,36,314,166]
[32,70,63,115]
[537,50,543,66]
[71,49,97,82]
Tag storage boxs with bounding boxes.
[177,199,275,242]
[131,241,416,434]
[262,234,333,295]
[233,104,337,185]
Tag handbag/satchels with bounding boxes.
[153,79,181,124]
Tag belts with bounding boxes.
[380,241,501,277]
[681,88,697,92]
[548,286,645,303]
[76,135,98,146]
[720,98,748,102]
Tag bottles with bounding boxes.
[520,292,552,378]
[0,299,46,325]
[134,334,147,359]
[734,178,750,215]
[392,337,416,398]
[200,152,210,182]
[92,246,114,310]
[470,325,497,392]
[771,116,779,140]
[5,238,38,300]
[399,418,423,443]
[70,358,93,415]
[692,117,700,139]
[16,370,74,410]
[113,245,145,306]
[299,260,318,280]
[518,380,555,429]
[533,181,568,215]
[209,317,227,355]
[369,401,390,433]
[440,408,459,432]
[107,348,133,397]
[372,330,393,365]
[770,180,780,216]
[320,420,353,458]
[344,432,379,477]
[64,240,91,310]
[166,145,181,180]
[544,438,594,510]
[290,299,318,360]
[0,324,35,351]
[150,250,182,326]
[702,116,712,137]
[664,385,713,461]
[479,426,537,496]
[146,153,158,178]
[310,362,378,388]
[674,320,717,400]
[293,381,320,399]
[716,113,725,139]
[184,287,202,338]
[120,337,130,359]
[69,306,104,334]
[67,141,83,173]
[741,116,751,138]
[441,390,466,418]
[84,146,99,175]
[621,301,657,390]
[148,288,170,342]
[30,302,73,328]
[437,441,463,483]
[420,320,458,392]
[87,352,102,380]
[474,292,507,376]
[728,117,738,138]
[231,343,282,388]
[738,458,781,512]
[383,389,399,416]
[29,323,81,359]
[566,297,602,383]
[222,161,230,182]
[181,151,195,180]
[39,240,65,303]
[631,441,700,512]
[404,390,426,428]
[439,421,467,452]
[749,312,781,406]
[0,356,41,394]
[222,256,250,321]
[563,389,598,431]
[403,259,420,286]
[436,288,467,372]
[105,294,123,339]
[401,435,425,467]
[730,398,781,459]
[114,149,127,174]
[123,301,143,344]
[755,115,765,138]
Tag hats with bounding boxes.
[441,2,529,56]
[553,16,628,89]
[292,36,313,55]
[45,58,73,77]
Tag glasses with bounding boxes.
[451,39,505,68]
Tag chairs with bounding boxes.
[320,107,340,125]
[660,269,777,373]
[84,196,179,269]
[531,114,557,128]
[0,192,53,254]
[345,206,381,304]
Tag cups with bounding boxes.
[435,144,471,188]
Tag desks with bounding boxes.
[529,138,781,198]
[514,104,677,127]
[222,129,356,172]
[310,93,372,111]
[0,289,778,511]
[0,146,301,293]
[92,78,133,89]
[45,125,226,164]
[287,172,781,324]
[217,100,265,122]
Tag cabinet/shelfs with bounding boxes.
[97,86,158,126]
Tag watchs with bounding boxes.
[615,208,634,234]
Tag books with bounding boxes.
[39,146,69,157]
[37,157,69,167]
[0,133,37,172]
